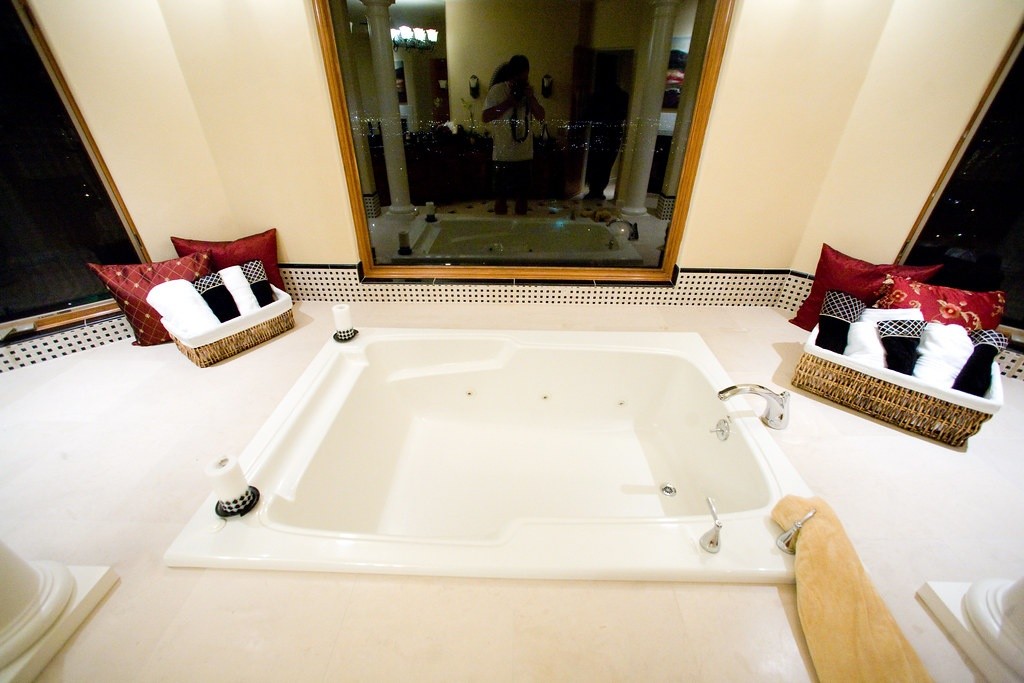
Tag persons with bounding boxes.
[580,65,628,208]
[483,54,542,216]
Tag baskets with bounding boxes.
[790,323,1004,448]
[160,283,296,368]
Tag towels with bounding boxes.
[240,258,279,308]
[815,290,866,359]
[215,264,263,317]
[861,304,927,326]
[913,324,973,393]
[770,494,931,682]
[191,273,241,324]
[146,278,222,338]
[877,320,929,377]
[952,327,1013,400]
[846,318,890,372]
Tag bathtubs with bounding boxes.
[392,209,647,271]
[164,322,822,584]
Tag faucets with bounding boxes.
[605,216,641,242]
[715,384,795,436]
